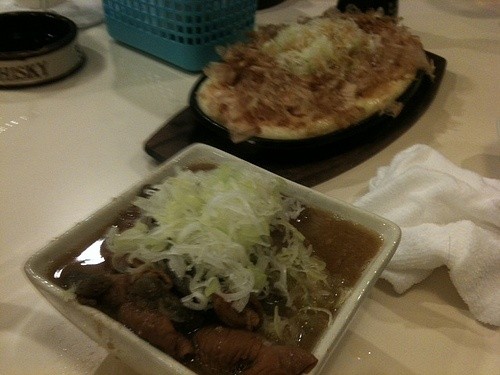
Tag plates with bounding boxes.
[189,57,429,145]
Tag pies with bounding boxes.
[195,10,432,142]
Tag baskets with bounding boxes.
[102,0,255,71]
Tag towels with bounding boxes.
[351,144,500,329]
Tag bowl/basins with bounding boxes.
[0,11,86,87]
[23,143,402,375]
